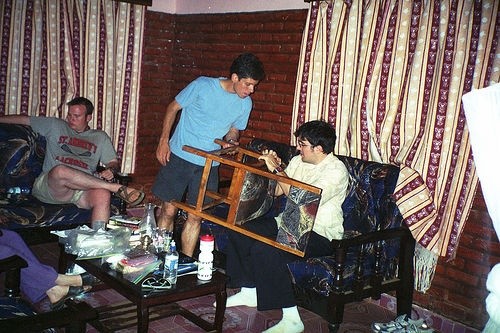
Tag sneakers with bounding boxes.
[371,313,436,333]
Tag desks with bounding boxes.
[56,242,231,332]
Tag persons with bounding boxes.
[208,120,349,333]
[150,52,266,258]
[0,231,104,310]
[0,97,144,231]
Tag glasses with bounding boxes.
[298,140,315,146]
[141,277,172,289]
[66,111,89,119]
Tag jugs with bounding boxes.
[140,204,157,242]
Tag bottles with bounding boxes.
[197,233,214,282]
[164,240,179,285]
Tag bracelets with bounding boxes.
[226,139,237,143]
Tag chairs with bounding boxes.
[0,255,99,333]
[169,139,322,258]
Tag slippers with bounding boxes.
[49,285,93,312]
[113,185,145,206]
[79,271,104,286]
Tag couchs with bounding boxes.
[173,138,416,333]
[0,123,133,231]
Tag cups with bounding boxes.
[93,220,105,231]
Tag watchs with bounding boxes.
[271,166,285,174]
